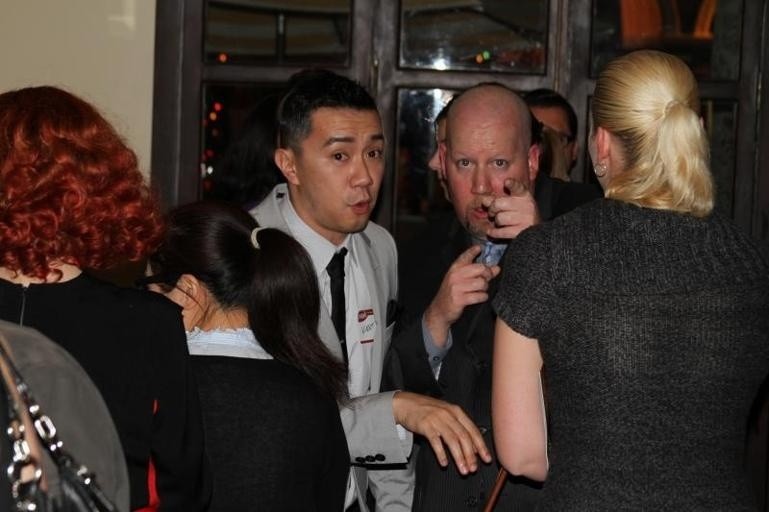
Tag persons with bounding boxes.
[523,89,580,179]
[0,87,212,512]
[428,94,459,203]
[0,320,131,512]
[248,72,490,512]
[492,50,769,512]
[145,200,351,512]
[381,85,603,512]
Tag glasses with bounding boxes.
[551,132,576,149]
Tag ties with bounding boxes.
[326,246,350,384]
